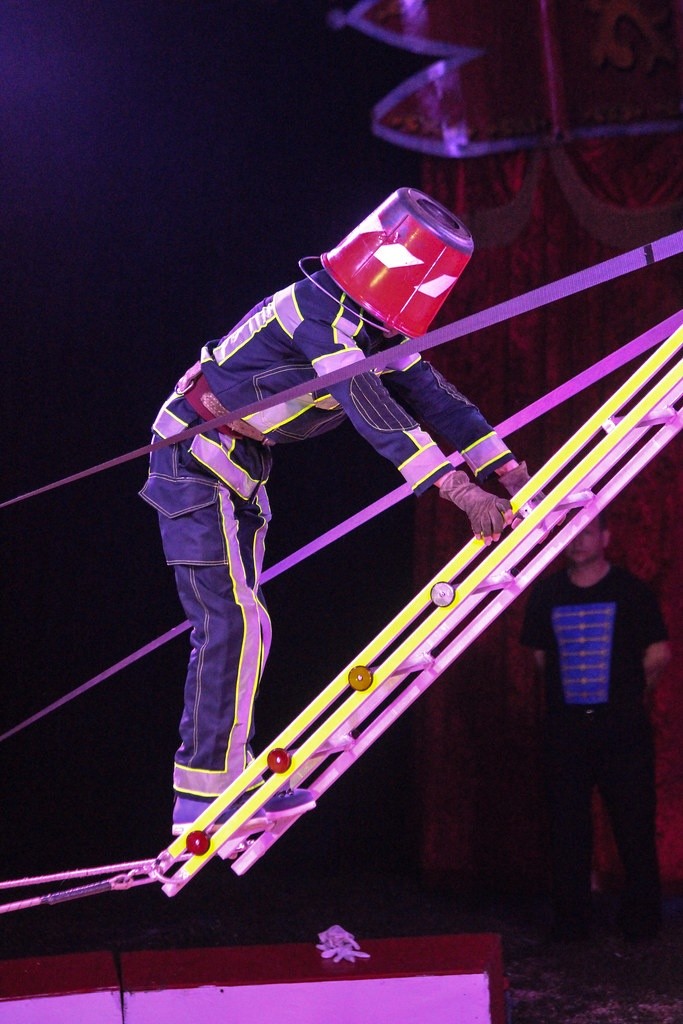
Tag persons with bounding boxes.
[138,186,566,836]
[519,504,674,940]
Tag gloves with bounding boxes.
[316,925,371,963]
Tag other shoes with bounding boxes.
[619,903,660,943]
[550,905,592,943]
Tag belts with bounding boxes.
[200,393,276,446]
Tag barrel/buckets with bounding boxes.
[297,189,474,339]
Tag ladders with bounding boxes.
[147,325,683,897]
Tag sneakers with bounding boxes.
[172,791,268,837]
[248,788,317,818]
